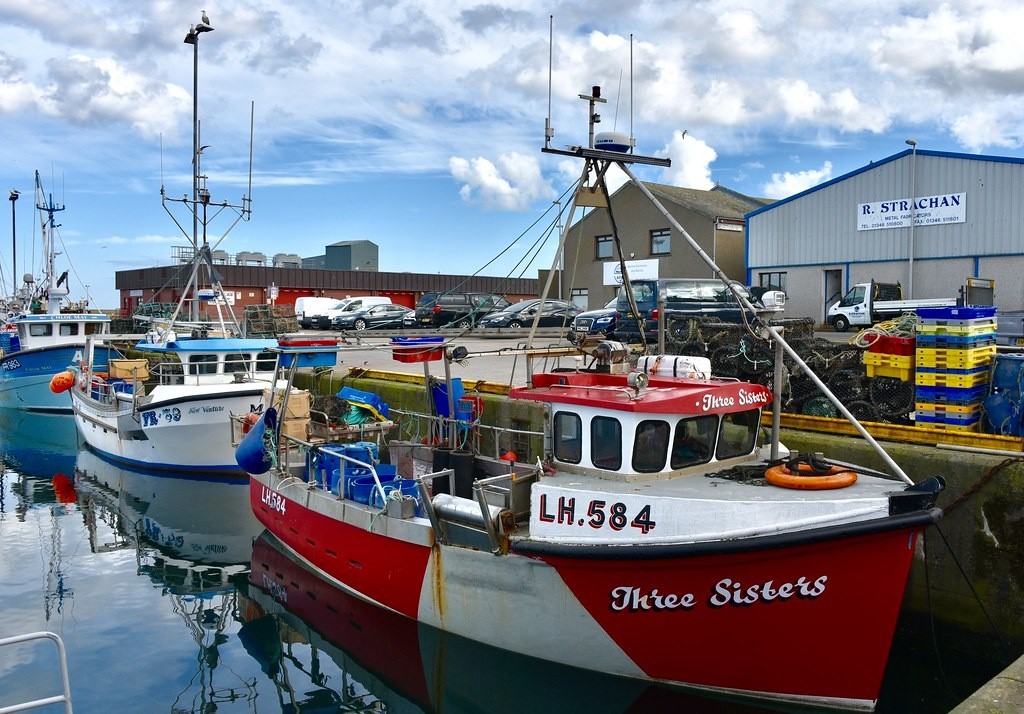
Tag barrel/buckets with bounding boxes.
[81,372,135,403]
[981,351,1024,438]
[304,440,425,518]
[430,378,475,435]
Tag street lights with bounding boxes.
[905,140,917,299]
[184,22,215,322]
[553,201,564,300]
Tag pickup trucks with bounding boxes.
[827,278,964,331]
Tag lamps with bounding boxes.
[760,290,786,309]
[627,372,649,401]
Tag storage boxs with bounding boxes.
[863,304,999,433]
[264,387,311,450]
[278,341,341,367]
[0,332,21,356]
[108,359,149,379]
[636,355,712,380]
[335,386,390,422]
[392,337,445,363]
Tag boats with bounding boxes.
[0,160,129,411]
[229,13,975,714]
[73,440,264,714]
[247,527,745,714]
[49,99,297,475]
[0,408,85,632]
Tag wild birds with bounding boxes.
[682,129,688,139]
[201,10,210,25]
[190,23,196,35]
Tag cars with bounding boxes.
[480,298,581,329]
[331,303,412,330]
[294,297,340,330]
[571,295,620,345]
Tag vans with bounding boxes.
[613,277,766,344]
[310,294,392,331]
[415,290,514,328]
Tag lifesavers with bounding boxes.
[81,376,110,400]
[243,414,260,439]
[246,602,266,623]
[764,464,858,491]
